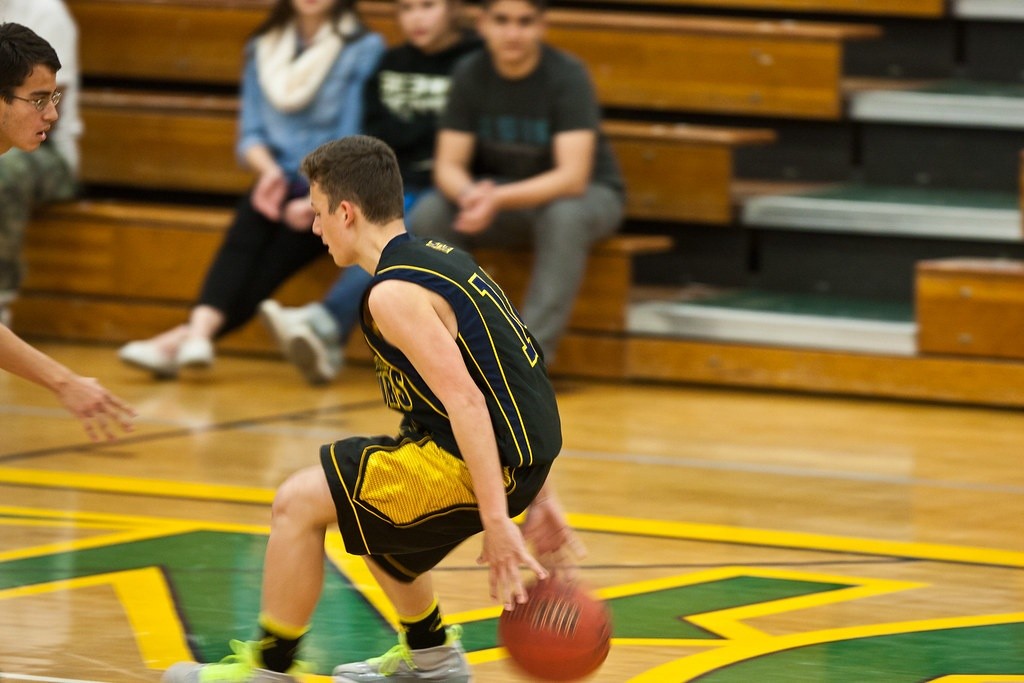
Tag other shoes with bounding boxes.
[117,340,176,379]
[286,326,335,383]
[260,298,294,357]
[177,335,212,367]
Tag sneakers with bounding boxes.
[161,638,317,683]
[332,624,471,683]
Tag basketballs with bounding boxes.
[499,580,614,683]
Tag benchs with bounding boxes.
[1,0,1024,411]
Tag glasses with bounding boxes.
[3,89,62,112]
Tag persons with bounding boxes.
[258,0,486,385]
[159,135,586,682]
[117,0,390,381]
[411,0,626,369]
[0,0,141,445]
